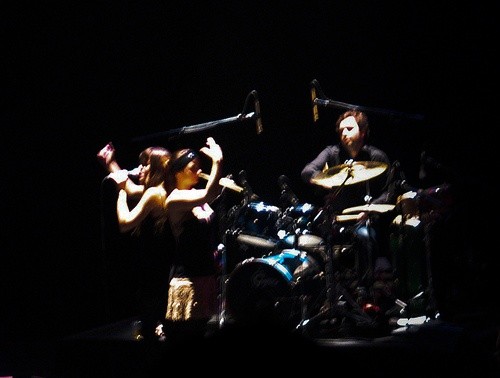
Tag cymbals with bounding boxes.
[311,161,389,187]
[201,172,244,195]
[340,203,396,217]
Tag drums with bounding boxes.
[237,200,279,248]
[280,206,327,248]
[230,248,330,336]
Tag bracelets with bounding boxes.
[105,158,117,170]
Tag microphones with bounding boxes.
[253,91,263,134]
[311,81,318,123]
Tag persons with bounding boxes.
[97,141,170,338]
[136,136,224,345]
[300,109,393,315]
